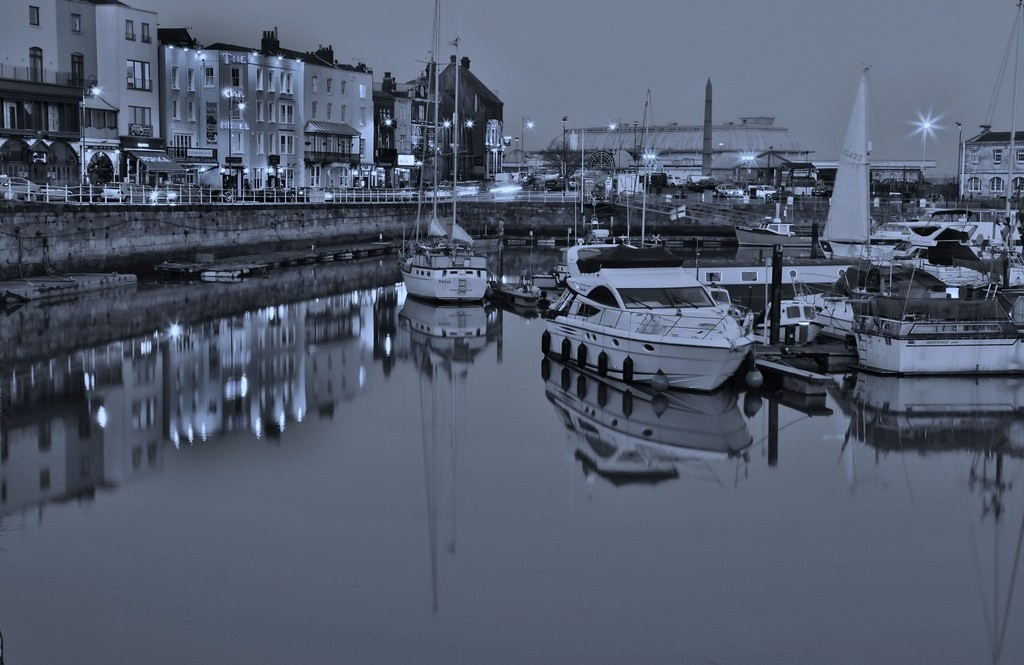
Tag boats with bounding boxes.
[839,370,1024,526]
[489,1,1024,394]
[540,354,755,491]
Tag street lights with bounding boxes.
[609,115,621,174]
[514,137,520,172]
[82,73,103,200]
[224,88,246,190]
[375,117,392,187]
[922,119,931,198]
[954,120,963,202]
[561,115,568,196]
[633,120,638,173]
[443,121,452,172]
[520,116,535,172]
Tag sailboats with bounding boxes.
[395,1,492,306]
[397,291,500,618]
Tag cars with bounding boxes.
[755,184,778,199]
[256,182,352,203]
[715,183,745,200]
[668,175,685,189]
[0,173,238,204]
[811,185,833,198]
